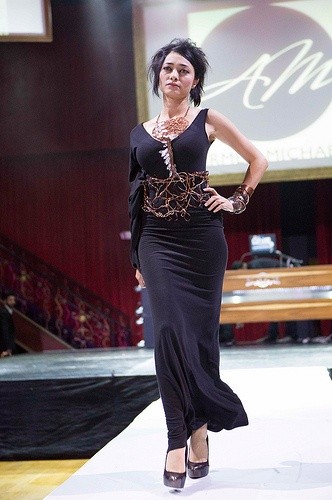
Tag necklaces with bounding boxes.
[151,106,192,142]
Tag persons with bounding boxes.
[128,37,271,490]
[0,295,16,358]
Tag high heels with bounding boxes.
[188,433,209,479]
[163,442,188,488]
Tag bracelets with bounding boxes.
[227,184,255,214]
